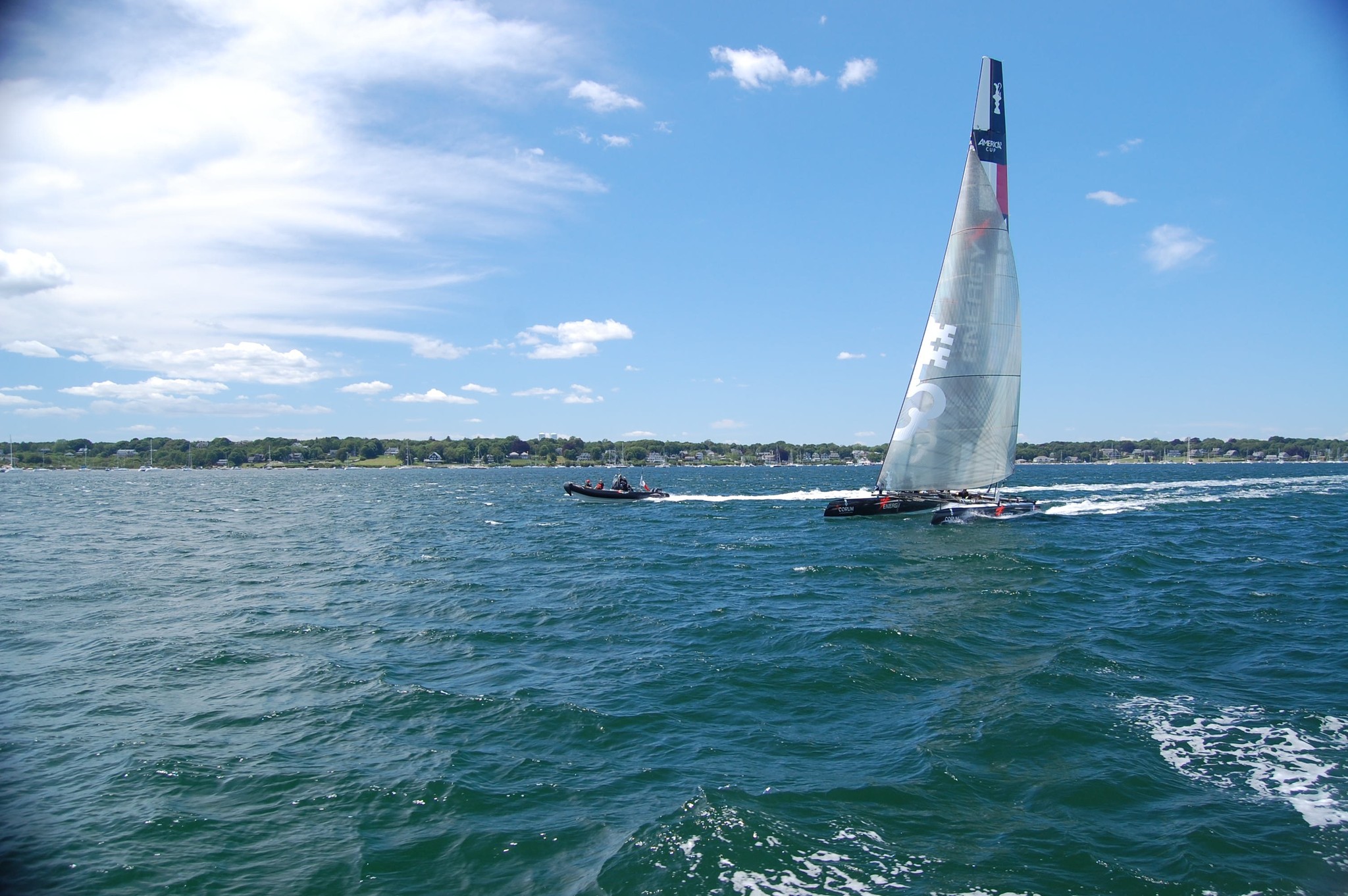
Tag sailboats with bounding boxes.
[823,55,1043,525]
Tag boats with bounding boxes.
[220,453,229,470]
[105,466,129,471]
[845,450,883,467]
[78,445,92,472]
[563,464,670,504]
[1107,436,1255,465]
[594,442,635,469]
[685,451,713,467]
[740,461,756,467]
[139,438,162,472]
[33,448,51,472]
[0,434,23,473]
[182,444,194,471]
[232,466,242,469]
[1275,448,1285,464]
[1307,445,1345,464]
[261,444,273,469]
[278,467,285,469]
[457,445,594,470]
[763,444,805,468]
[344,453,365,470]
[399,440,413,469]
[655,448,670,468]
[306,465,319,470]
[1058,451,1065,465]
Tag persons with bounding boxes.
[584,480,592,487]
[620,476,627,490]
[955,489,969,500]
[594,479,605,490]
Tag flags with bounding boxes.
[641,475,650,491]
